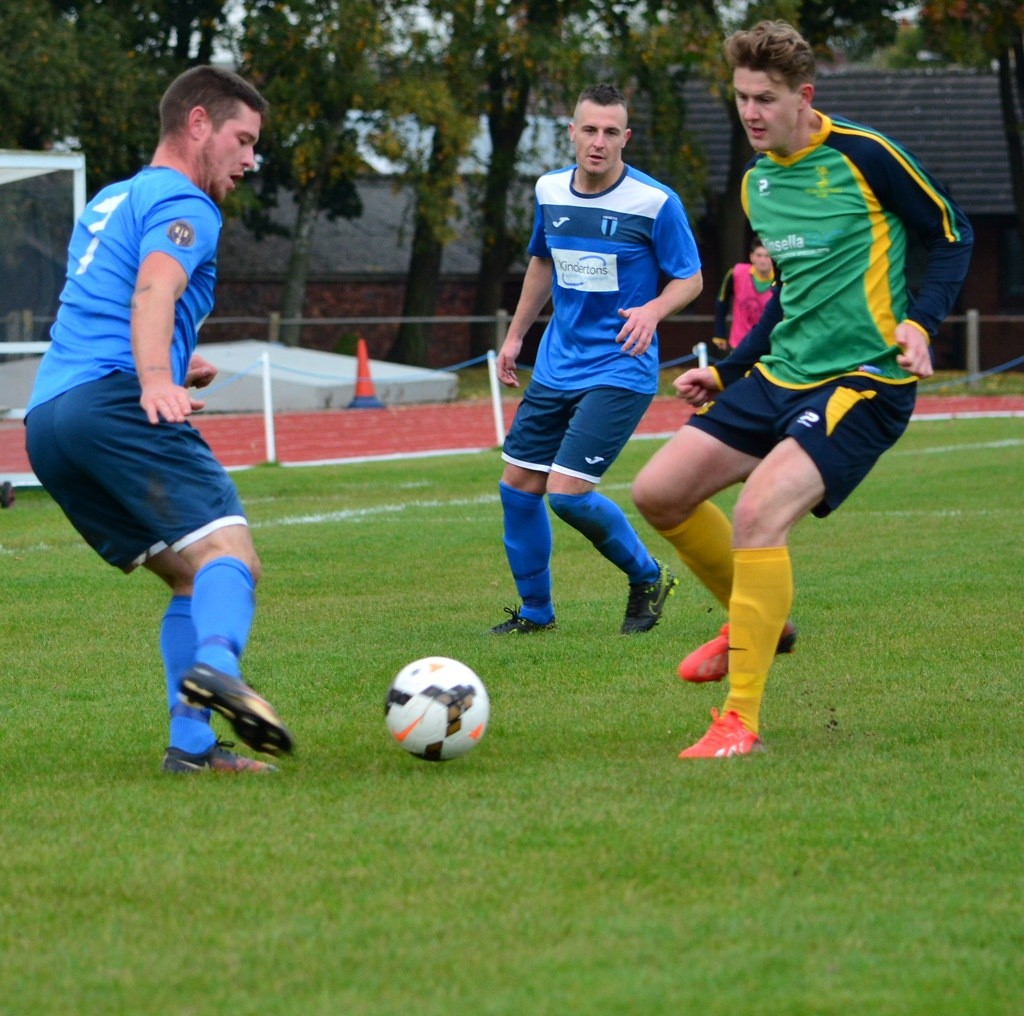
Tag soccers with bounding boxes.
[383,655,492,763]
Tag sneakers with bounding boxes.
[177,661,290,755]
[493,604,555,635]
[164,737,279,778]
[680,623,796,684]
[678,707,766,758]
[621,558,680,636]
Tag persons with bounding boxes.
[711,237,775,358]
[23,65,295,772]
[631,19,974,758]
[490,84,703,635]
